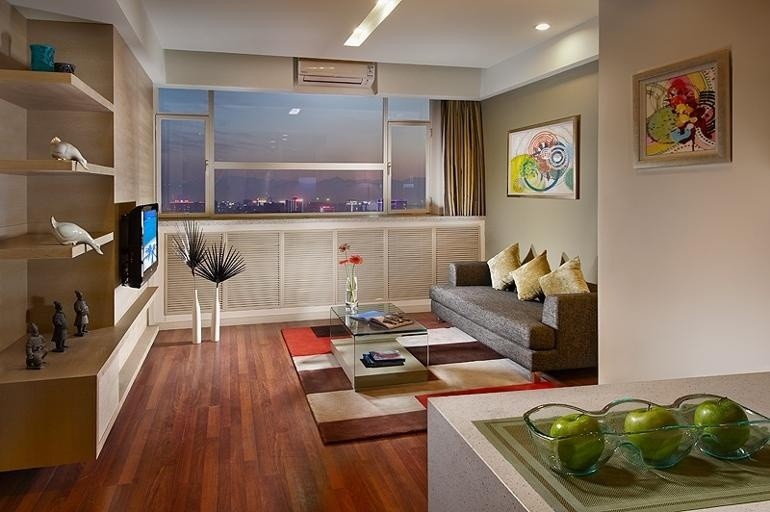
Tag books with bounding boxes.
[348,310,414,329]
[360,350,406,367]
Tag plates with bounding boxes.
[524,395,770,477]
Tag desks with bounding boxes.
[429,372,769,512]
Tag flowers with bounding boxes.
[338,241,364,277]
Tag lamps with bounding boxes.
[534,23,550,31]
[344,1,401,48]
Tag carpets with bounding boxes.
[281,319,600,446]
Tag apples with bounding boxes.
[694,396,751,453]
[550,412,606,469]
[624,403,683,460]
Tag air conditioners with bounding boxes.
[292,58,376,89]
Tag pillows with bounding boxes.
[487,243,522,290]
[539,254,590,298]
[510,250,551,302]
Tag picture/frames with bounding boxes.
[506,115,581,201]
[632,49,733,170]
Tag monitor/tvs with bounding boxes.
[125,203,159,290]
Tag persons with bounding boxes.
[52,300,69,353]
[74,290,89,336]
[26,322,49,370]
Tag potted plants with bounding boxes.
[170,216,206,345]
[194,241,246,342]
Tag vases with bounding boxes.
[345,276,360,310]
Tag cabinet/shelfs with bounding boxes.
[283,227,386,309]
[163,232,282,316]
[0,2,159,473]
[386,225,482,301]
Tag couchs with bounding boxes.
[429,260,598,382]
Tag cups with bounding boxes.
[56,62,75,74]
[30,44,56,72]
